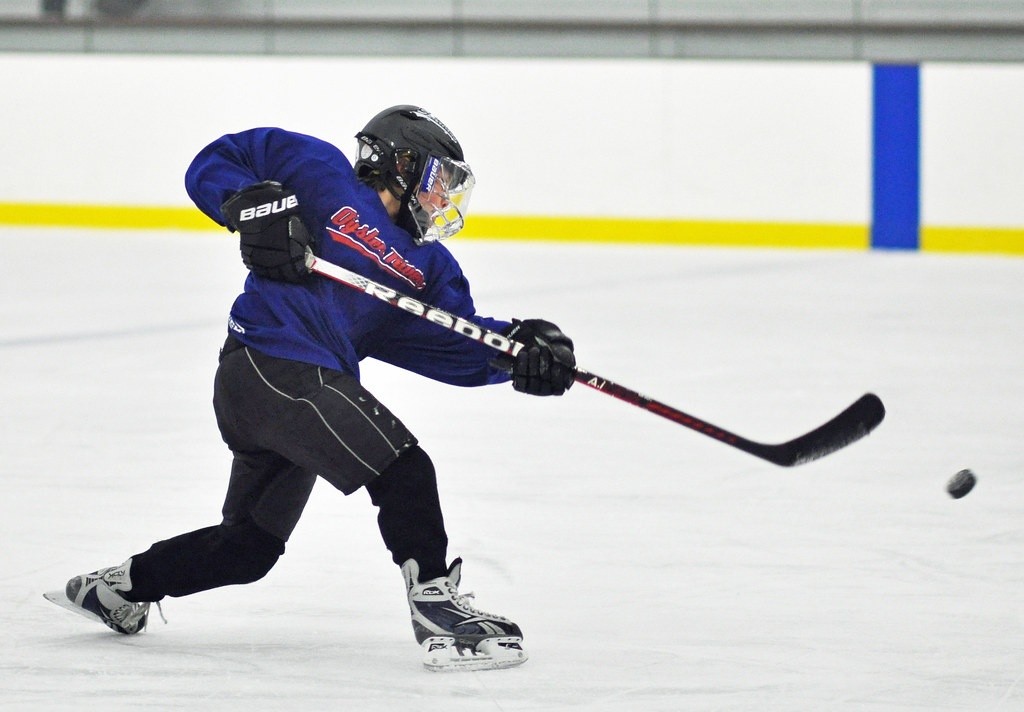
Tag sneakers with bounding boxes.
[400,557,540,673]
[43,559,169,635]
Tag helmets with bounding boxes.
[355,105,473,242]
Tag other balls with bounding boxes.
[946,468,978,500]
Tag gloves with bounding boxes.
[501,317,575,398]
[220,181,316,283]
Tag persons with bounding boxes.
[42,104,577,673]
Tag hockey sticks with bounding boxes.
[303,252,889,470]
